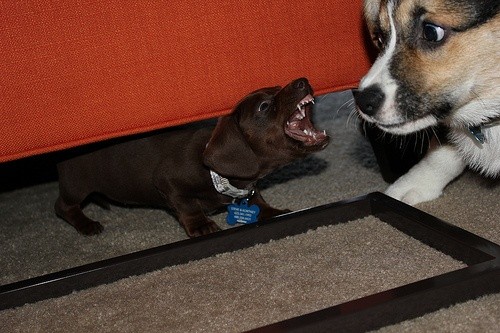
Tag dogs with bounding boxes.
[348,1,500,205]
[53,77,331,240]
